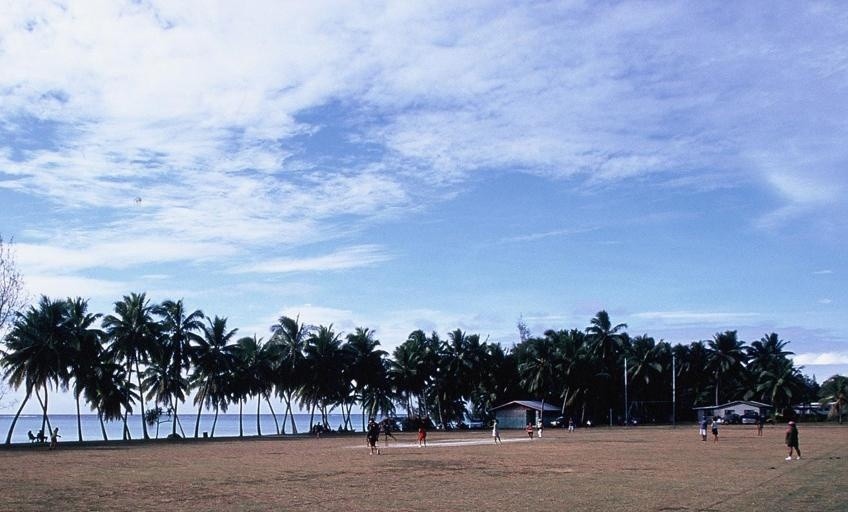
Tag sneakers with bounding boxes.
[785,456,800,460]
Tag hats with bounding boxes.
[788,421,795,425]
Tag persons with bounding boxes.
[383,422,398,441]
[525,422,533,439]
[417,427,427,448]
[756,416,763,436]
[584,415,592,432]
[489,419,502,445]
[698,415,708,441]
[565,417,576,432]
[711,416,719,444]
[365,418,381,455]
[28,427,61,450]
[784,420,801,461]
[536,419,544,438]
[313,422,351,435]
